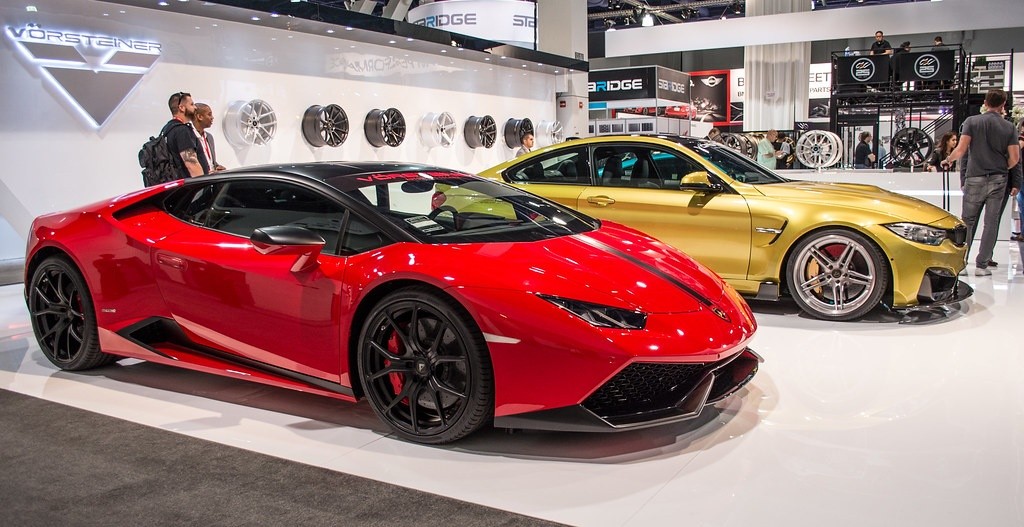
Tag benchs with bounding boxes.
[519,159,589,182]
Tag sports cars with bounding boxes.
[19,157,761,450]
[433,128,976,324]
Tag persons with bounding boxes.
[893,41,911,90]
[940,89,1019,276]
[771,133,794,169]
[757,129,783,170]
[515,133,535,158]
[879,140,886,161]
[870,31,892,92]
[189,103,225,174]
[160,92,207,177]
[704,128,720,140]
[855,131,875,168]
[930,131,958,172]
[960,107,1024,267]
[927,36,949,89]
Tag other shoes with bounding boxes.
[974,267,992,276]
[988,261,998,266]
[958,268,968,276]
[1011,232,1024,242]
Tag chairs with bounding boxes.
[601,156,623,186]
[629,159,650,187]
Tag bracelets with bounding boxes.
[946,156,952,164]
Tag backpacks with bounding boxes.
[138,122,194,187]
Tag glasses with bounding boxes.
[876,35,881,37]
[178,92,185,108]
